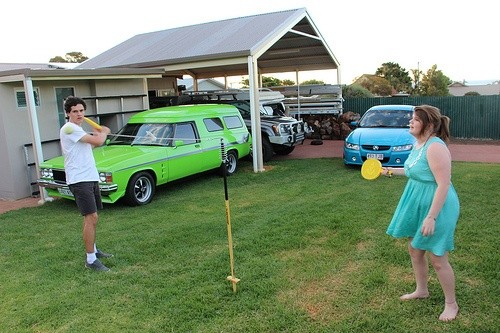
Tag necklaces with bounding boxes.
[407,134,431,168]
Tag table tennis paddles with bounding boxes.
[361,158,393,180]
[83,117,103,131]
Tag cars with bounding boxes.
[342,104,417,169]
[36,104,253,206]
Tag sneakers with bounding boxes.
[95,249,115,259]
[84,259,110,272]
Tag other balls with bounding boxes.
[64,124,74,135]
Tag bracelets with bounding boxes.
[386,166,394,178]
[427,215,436,222]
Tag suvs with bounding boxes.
[176,88,305,163]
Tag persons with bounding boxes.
[60,96,113,272]
[382,104,460,322]
